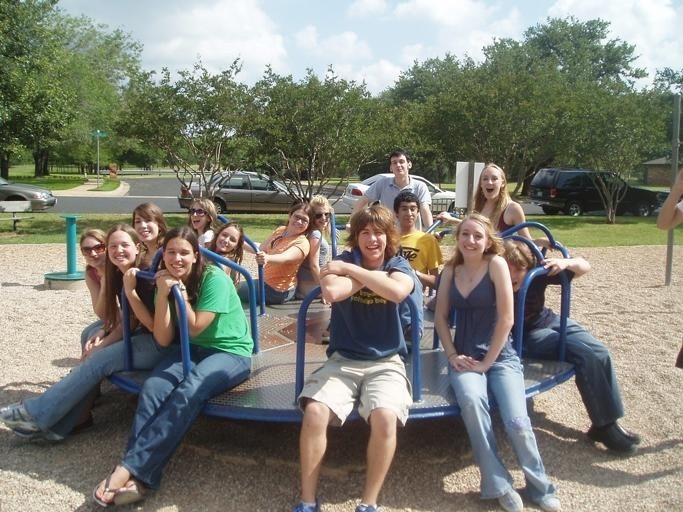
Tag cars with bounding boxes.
[0,177,59,214]
[178,172,317,216]
[341,171,456,215]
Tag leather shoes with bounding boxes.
[613,420,643,445]
[586,424,638,457]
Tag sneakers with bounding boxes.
[354,499,378,512]
[530,491,562,512]
[290,497,321,512]
[497,486,524,512]
[402,323,422,354]
[0,400,41,432]
[12,428,66,442]
[321,323,332,345]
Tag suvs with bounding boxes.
[528,166,660,216]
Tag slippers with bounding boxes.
[112,480,149,507]
[92,464,119,508]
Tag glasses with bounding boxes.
[80,244,106,257]
[187,208,208,217]
[314,213,331,220]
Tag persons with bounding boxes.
[436,216,564,512]
[290,206,418,512]
[499,239,642,454]
[348,148,440,241]
[656,162,683,370]
[90,227,253,506]
[438,162,554,253]
[0,221,166,444]
[70,195,447,358]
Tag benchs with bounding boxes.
[0,200,34,231]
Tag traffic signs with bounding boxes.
[98,131,108,137]
